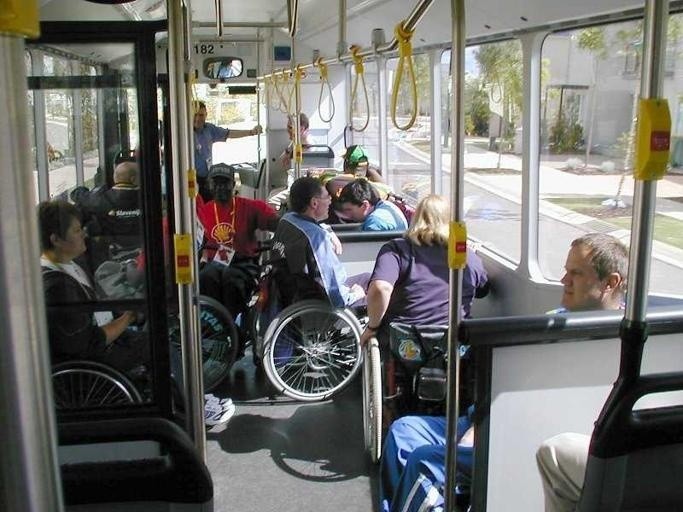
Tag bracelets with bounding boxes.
[249,130,254,137]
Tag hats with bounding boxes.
[209,163,235,182]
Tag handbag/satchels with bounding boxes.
[411,323,448,403]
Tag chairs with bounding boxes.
[281,140,682,512]
[65,148,144,277]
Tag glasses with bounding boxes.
[320,194,332,201]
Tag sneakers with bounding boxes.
[204,400,235,427]
[203,393,233,407]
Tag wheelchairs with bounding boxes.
[50,326,231,435]
[96,230,274,394]
[362,293,489,463]
[254,246,370,403]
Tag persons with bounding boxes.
[375,232,633,511]
[190,101,264,184]
[216,60,234,78]
[38,145,489,434]
[533,431,589,510]
[277,112,310,163]
